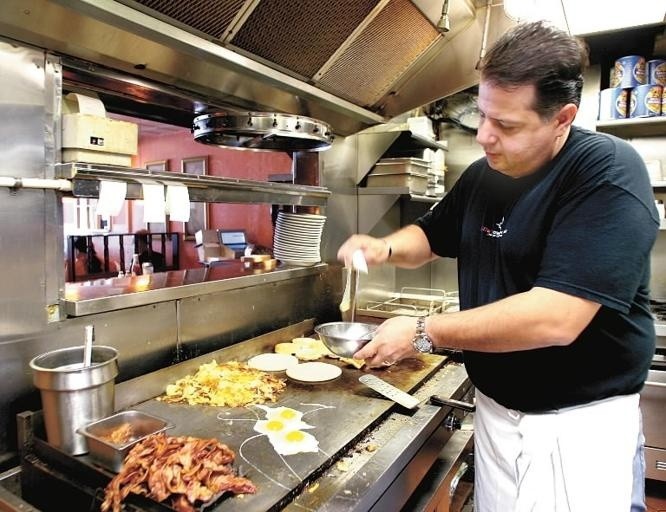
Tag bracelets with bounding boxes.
[381,237,391,260]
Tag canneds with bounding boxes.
[600,55,666,121]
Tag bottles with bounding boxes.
[118,254,154,279]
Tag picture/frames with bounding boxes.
[181,155,210,241]
[96,215,111,233]
[144,160,170,241]
[110,200,130,234]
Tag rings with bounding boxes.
[384,360,392,367]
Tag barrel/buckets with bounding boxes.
[30,346,120,456]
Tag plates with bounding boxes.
[245,350,299,371]
[273,211,326,267]
[283,359,344,385]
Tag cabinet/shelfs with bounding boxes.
[357,124,449,237]
[597,117,666,191]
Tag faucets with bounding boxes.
[129,254,137,274]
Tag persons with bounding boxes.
[62,237,119,282]
[128,229,165,273]
[335,19,660,511]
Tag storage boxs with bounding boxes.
[61,114,139,167]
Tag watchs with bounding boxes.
[412,316,434,355]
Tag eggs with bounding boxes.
[253,405,320,456]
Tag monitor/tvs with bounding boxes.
[218,229,246,251]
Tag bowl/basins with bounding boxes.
[315,321,378,358]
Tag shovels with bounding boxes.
[358,373,477,413]
